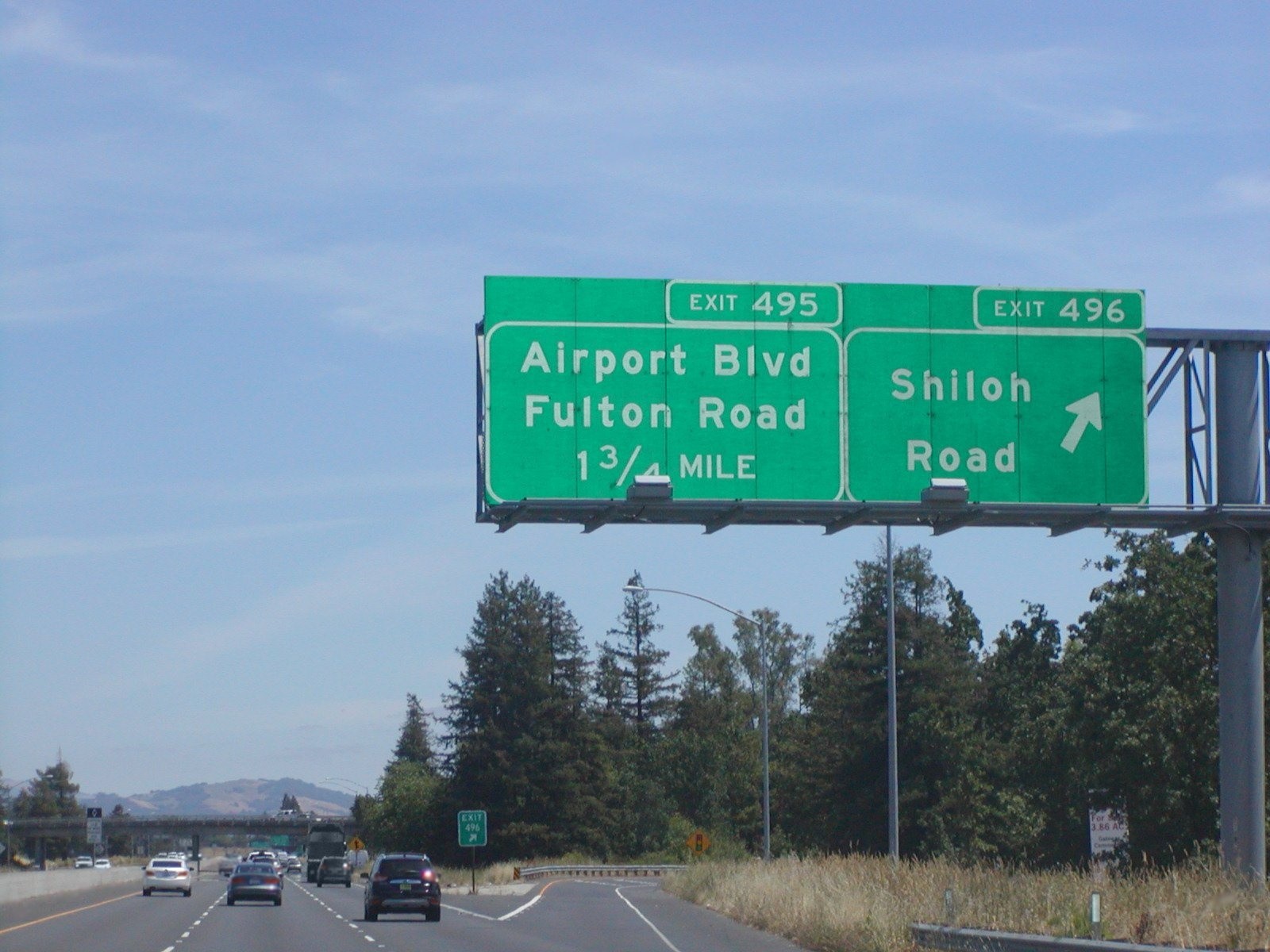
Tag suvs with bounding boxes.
[318,856,352,887]
[360,852,441,923]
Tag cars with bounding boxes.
[73,855,93,868]
[141,859,194,898]
[157,852,202,862]
[243,849,303,877]
[93,859,111,869]
[227,863,282,905]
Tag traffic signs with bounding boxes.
[483,273,846,503]
[837,283,1147,506]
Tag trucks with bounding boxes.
[306,821,347,882]
